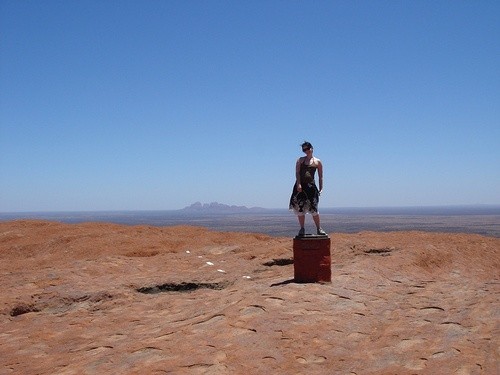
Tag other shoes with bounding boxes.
[299,228,305,235]
[317,227,326,235]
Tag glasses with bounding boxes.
[302,146,311,152]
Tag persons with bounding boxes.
[289,142,327,236]
